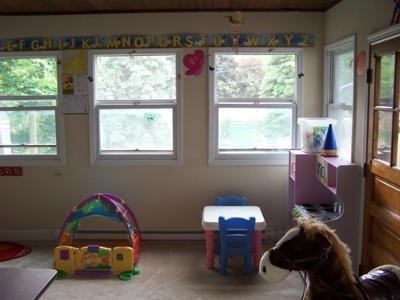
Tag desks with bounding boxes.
[203,205,267,269]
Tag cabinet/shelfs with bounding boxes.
[289,148,361,276]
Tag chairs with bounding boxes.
[215,195,255,274]
